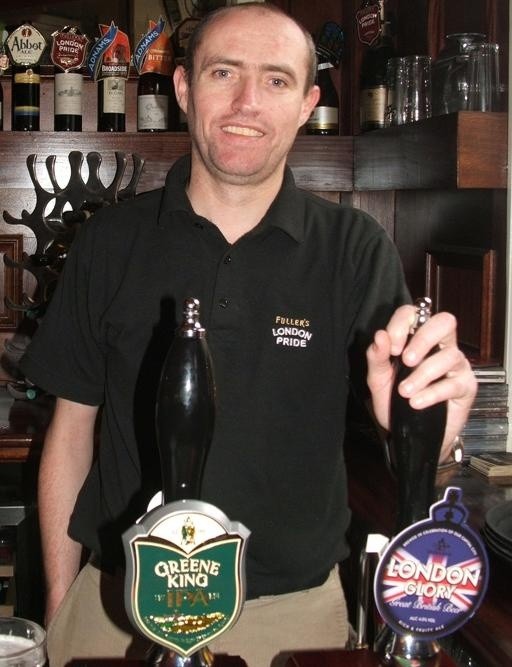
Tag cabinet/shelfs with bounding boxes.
[0,76,510,667]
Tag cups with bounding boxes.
[384,55,432,128]
[466,43,500,112]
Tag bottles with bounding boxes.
[11,19,171,132]
[306,1,396,135]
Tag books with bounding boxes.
[454,354,512,477]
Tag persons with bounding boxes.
[16,3,479,667]
